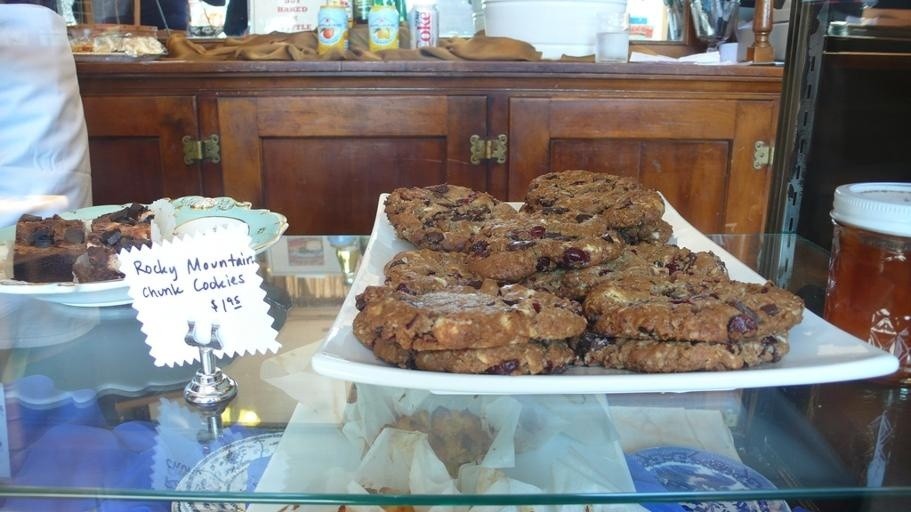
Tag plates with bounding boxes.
[305,188,900,396]
[633,444,793,512]
[0,193,290,308]
[164,428,286,512]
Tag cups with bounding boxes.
[592,10,630,66]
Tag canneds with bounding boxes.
[410,5,439,51]
[823,182,911,388]
[367,7,400,51]
[315,3,348,56]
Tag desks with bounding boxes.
[0,233,911,512]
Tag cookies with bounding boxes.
[352,170,805,376]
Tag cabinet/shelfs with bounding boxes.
[76,65,206,200]
[487,63,787,431]
[206,63,488,234]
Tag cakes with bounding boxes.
[14,202,155,281]
